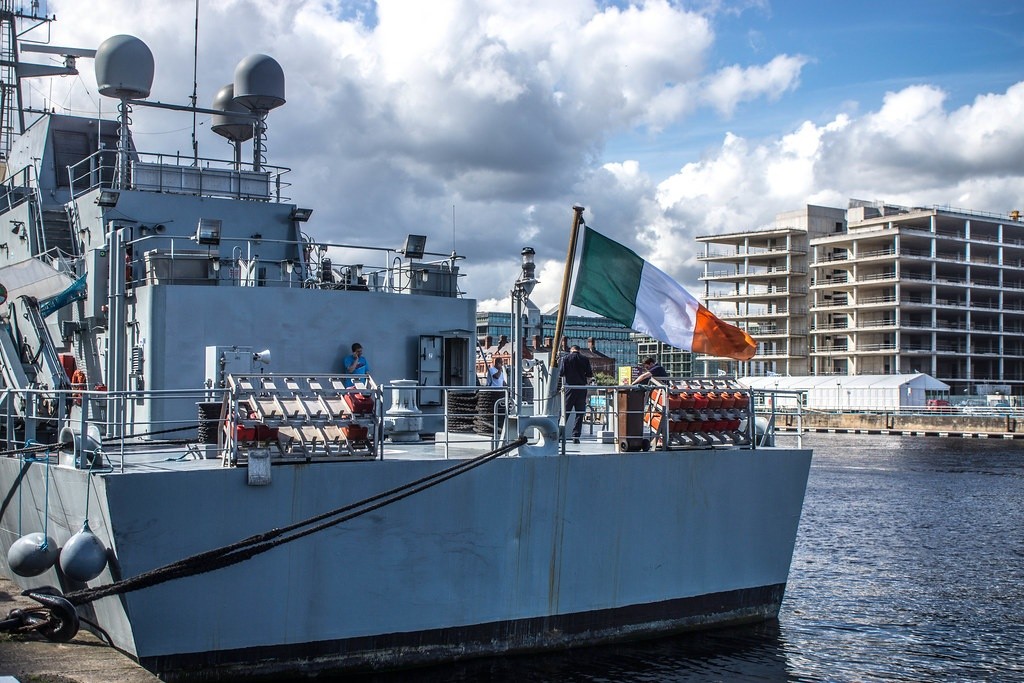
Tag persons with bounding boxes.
[631,358,671,389]
[487,357,506,386]
[557,345,593,443]
[344,343,372,390]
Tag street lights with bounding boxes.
[775,384,779,412]
[837,383,841,414]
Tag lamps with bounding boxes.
[188,217,222,245]
[288,209,314,222]
[395,234,427,259]
[96,191,120,207]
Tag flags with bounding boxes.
[570,225,759,361]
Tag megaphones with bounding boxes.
[522,358,538,372]
[253,350,271,364]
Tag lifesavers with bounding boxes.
[71,370,87,406]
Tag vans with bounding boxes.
[926,399,958,415]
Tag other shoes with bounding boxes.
[573,439,580,444]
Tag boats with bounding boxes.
[0,0,817,666]
